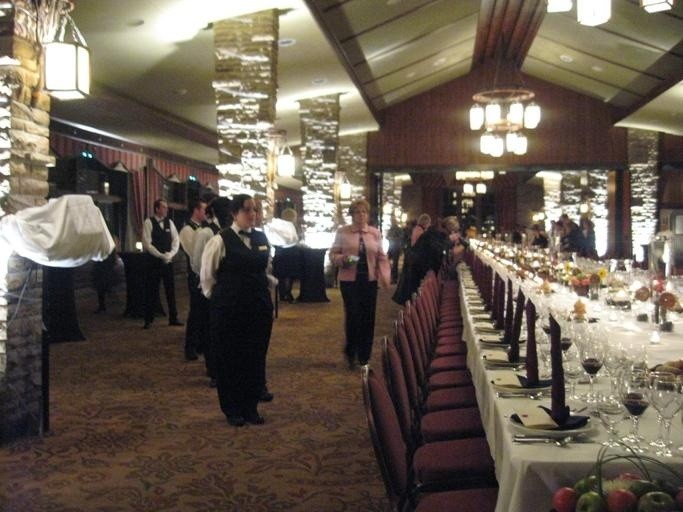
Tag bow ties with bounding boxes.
[238,229,254,238]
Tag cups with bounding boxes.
[649,371,675,447]
[620,378,653,453]
[580,343,607,400]
[652,378,683,456]
[556,333,572,367]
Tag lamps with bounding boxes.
[545,0,574,14]
[469,31,541,158]
[638,0,674,14]
[43,0,92,102]
[277,143,296,178]
[339,176,352,199]
[576,0,612,27]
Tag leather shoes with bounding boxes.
[226,414,245,426]
[259,389,273,402]
[142,322,152,330]
[247,411,265,424]
[168,318,184,326]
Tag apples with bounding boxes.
[637,491,674,512]
[607,489,636,512]
[575,491,605,512]
[552,487,576,512]
[575,475,596,496]
[629,480,656,498]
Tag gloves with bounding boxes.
[160,251,173,263]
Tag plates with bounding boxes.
[507,409,594,435]
[479,337,506,347]
[490,373,552,393]
[481,354,525,366]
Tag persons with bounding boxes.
[141,198,186,330]
[510,214,600,262]
[410,212,431,248]
[190,196,219,275]
[200,194,276,427]
[391,216,461,308]
[178,198,209,361]
[328,200,391,368]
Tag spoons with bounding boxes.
[497,393,544,399]
[513,436,581,448]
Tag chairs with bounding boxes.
[380,335,484,451]
[398,309,477,398]
[416,286,467,346]
[421,284,462,337]
[392,318,477,418]
[404,299,467,375]
[411,291,472,358]
[360,364,494,512]
[421,283,462,330]
